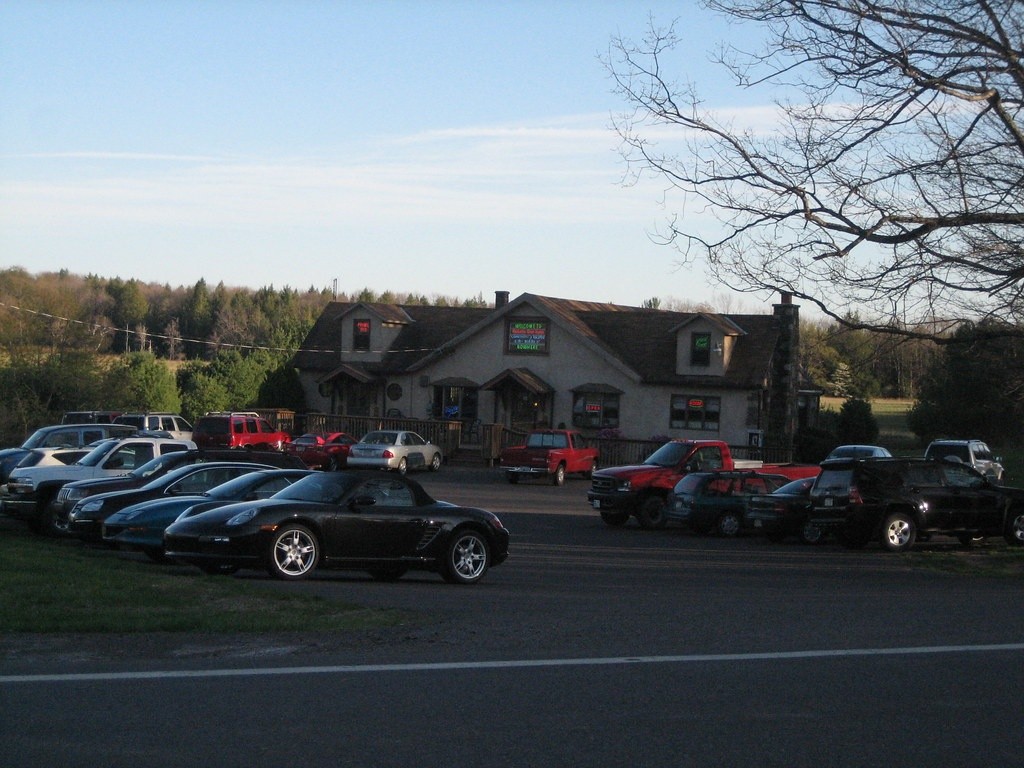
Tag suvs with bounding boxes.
[54,447,312,550]
[809,454,1024,554]
[924,437,1005,487]
[0,411,208,529]
[661,471,796,540]
[191,409,292,452]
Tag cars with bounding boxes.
[820,443,891,470]
[741,476,820,547]
[285,431,359,472]
[99,468,325,576]
[66,462,292,553]
[346,429,444,477]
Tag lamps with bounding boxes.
[713,340,723,358]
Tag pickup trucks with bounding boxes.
[499,429,601,486]
[587,438,822,531]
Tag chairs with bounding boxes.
[752,485,761,494]
[133,449,150,471]
[746,484,753,494]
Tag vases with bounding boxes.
[602,450,623,468]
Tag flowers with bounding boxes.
[597,428,626,451]
[637,434,671,460]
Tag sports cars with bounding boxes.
[163,471,510,583]
